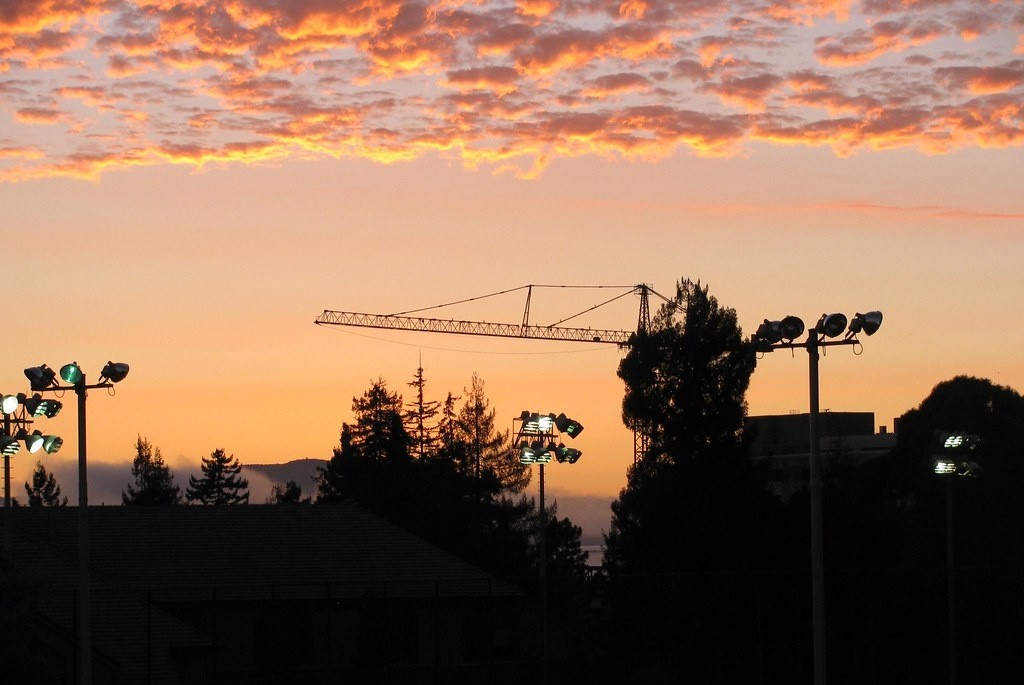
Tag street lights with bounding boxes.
[518,412,584,506]
[0,393,63,506]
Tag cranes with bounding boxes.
[313,283,691,469]
